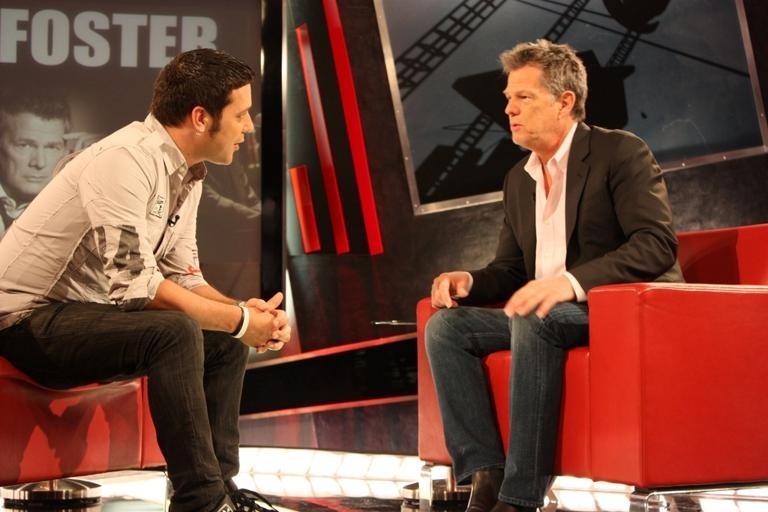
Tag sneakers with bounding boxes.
[217,476,278,512]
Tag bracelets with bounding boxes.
[232,305,250,340]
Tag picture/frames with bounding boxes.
[1,1,287,315]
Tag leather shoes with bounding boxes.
[464,468,539,512]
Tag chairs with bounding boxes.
[402,224,768,512]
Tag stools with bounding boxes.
[1,351,166,503]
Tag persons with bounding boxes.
[0,50,297,512]
[424,41,688,511]
[0,79,104,249]
